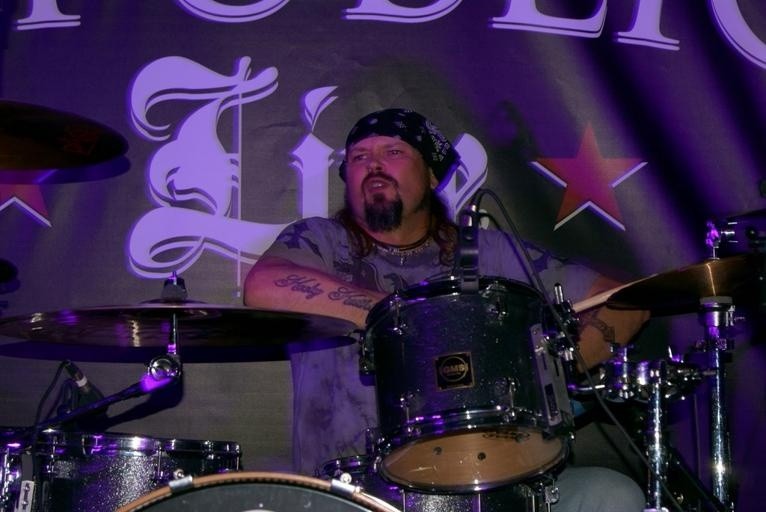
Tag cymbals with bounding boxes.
[0,298,359,347]
[606,251,765,308]
[727,208,764,220]
[2,99,130,170]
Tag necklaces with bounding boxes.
[357,210,436,259]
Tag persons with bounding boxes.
[239,107,656,512]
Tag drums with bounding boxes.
[1,425,244,512]
[315,454,559,511]
[358,275,576,494]
[109,472,402,511]
